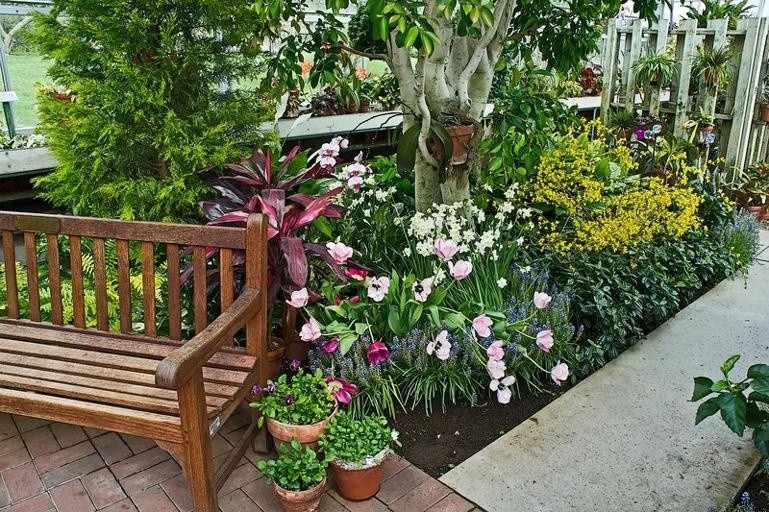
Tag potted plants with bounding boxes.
[433,110,483,166]
[338,70,377,114]
[179,142,346,405]
[760,73,769,123]
[689,42,738,90]
[682,105,716,145]
[628,47,679,90]
[722,160,769,223]
[255,408,395,511]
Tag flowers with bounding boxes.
[249,358,359,429]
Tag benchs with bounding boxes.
[0,210,268,511]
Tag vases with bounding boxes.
[265,406,339,457]
